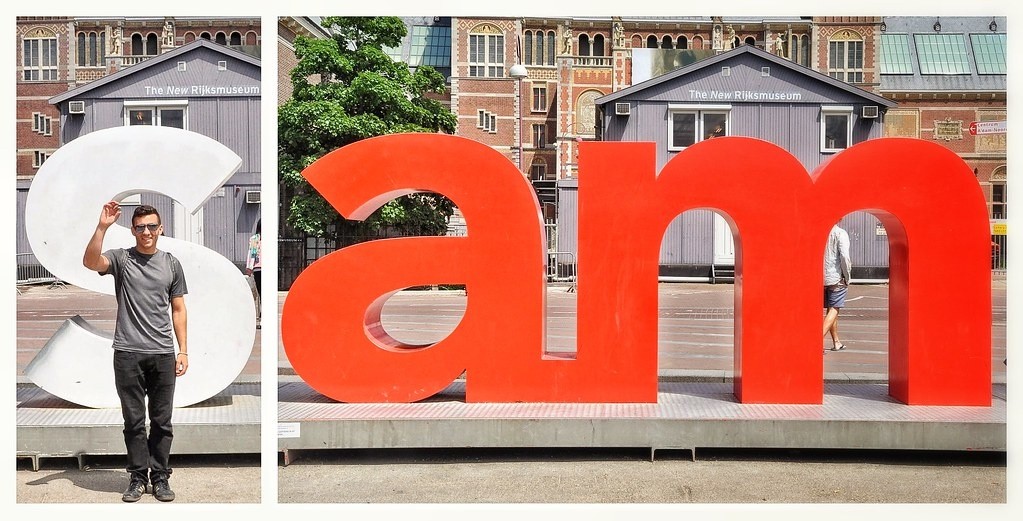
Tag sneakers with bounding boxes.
[152,478,175,501]
[121,478,148,502]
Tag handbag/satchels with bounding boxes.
[245,275,258,302]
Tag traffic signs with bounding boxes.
[969,120,1007,135]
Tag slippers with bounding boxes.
[833,344,846,351]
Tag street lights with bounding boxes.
[511,35,529,171]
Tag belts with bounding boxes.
[253,268,261,272]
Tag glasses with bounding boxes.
[133,224,159,232]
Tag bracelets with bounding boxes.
[177,352,188,357]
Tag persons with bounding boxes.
[83,200,188,501]
[562,28,572,55]
[726,25,736,49]
[111,29,121,55]
[822,217,851,351]
[715,29,721,49]
[615,23,621,47]
[162,21,169,45]
[246,218,261,328]
[776,33,785,57]
